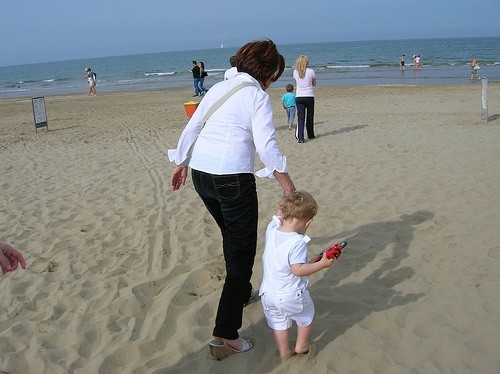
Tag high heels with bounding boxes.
[243,288,256,308]
[208,336,253,361]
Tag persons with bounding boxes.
[168,38,297,360]
[83,67,99,97]
[188,60,210,98]
[400,55,406,71]
[282,84,296,130]
[413,54,422,70]
[293,55,317,143]
[470,55,481,81]
[259,190,334,361]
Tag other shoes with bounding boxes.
[192,95,198,97]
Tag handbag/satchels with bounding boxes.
[203,72,208,76]
